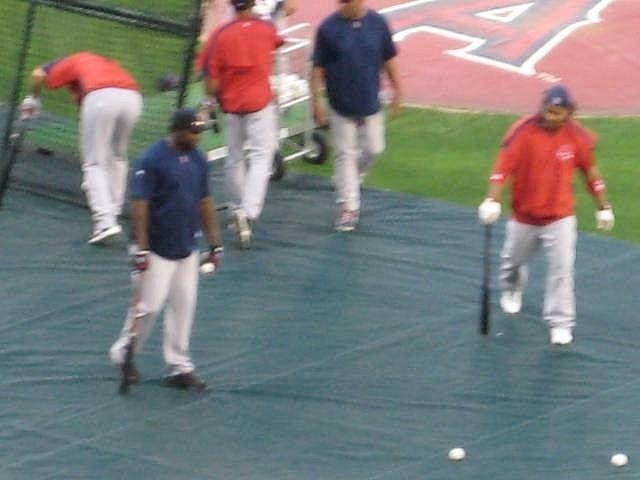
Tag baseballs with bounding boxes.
[199,264,214,276]
[449,446,464,463]
[269,73,310,107]
[611,455,628,468]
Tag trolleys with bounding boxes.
[267,22,327,179]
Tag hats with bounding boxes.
[170,110,198,129]
[543,86,571,109]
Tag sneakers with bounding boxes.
[88,224,122,244]
[165,371,208,390]
[122,362,138,382]
[500,289,523,314]
[550,324,574,344]
[231,209,252,250]
[334,209,361,232]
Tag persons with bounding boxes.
[477,87,615,346]
[202,0,286,250]
[310,1,404,235]
[32,48,145,248]
[104,108,222,391]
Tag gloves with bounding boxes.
[596,207,615,233]
[477,197,502,226]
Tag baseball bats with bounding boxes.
[118,273,141,392]
[1,93,39,206]
[479,222,489,335]
